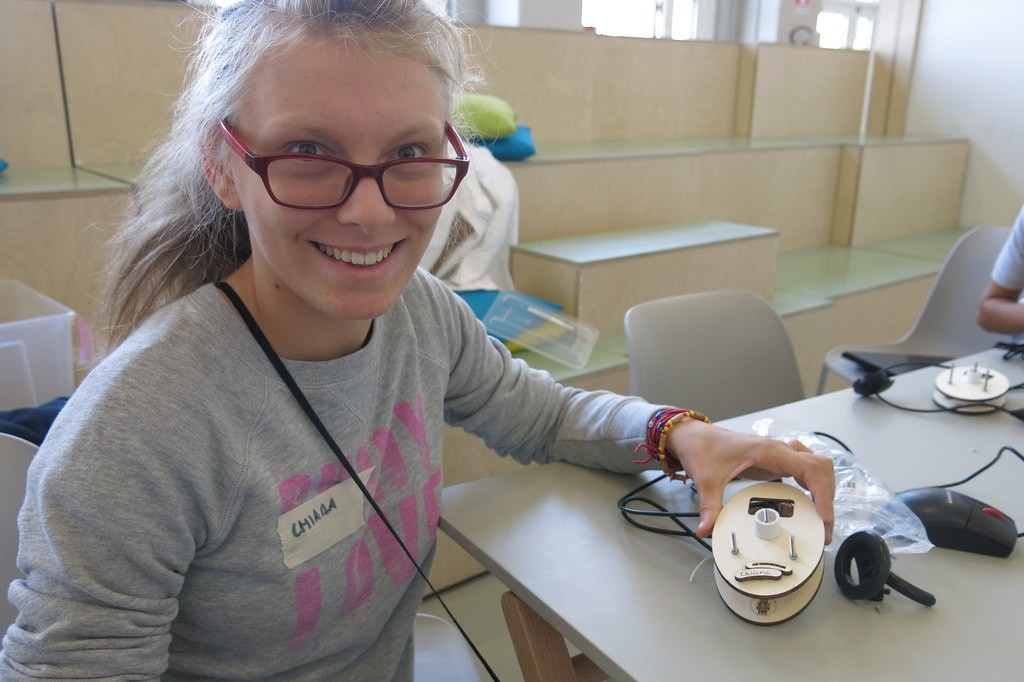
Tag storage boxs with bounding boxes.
[0,275,78,412]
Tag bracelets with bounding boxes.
[634,408,710,482]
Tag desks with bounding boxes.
[437,344,1024,682]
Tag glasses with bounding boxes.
[215,119,471,210]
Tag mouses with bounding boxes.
[895,487,1017,559]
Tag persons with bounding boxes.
[0,0,836,682]
[977,206,1024,334]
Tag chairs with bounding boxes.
[624,289,805,424]
[0,432,483,682]
[818,224,1013,395]
